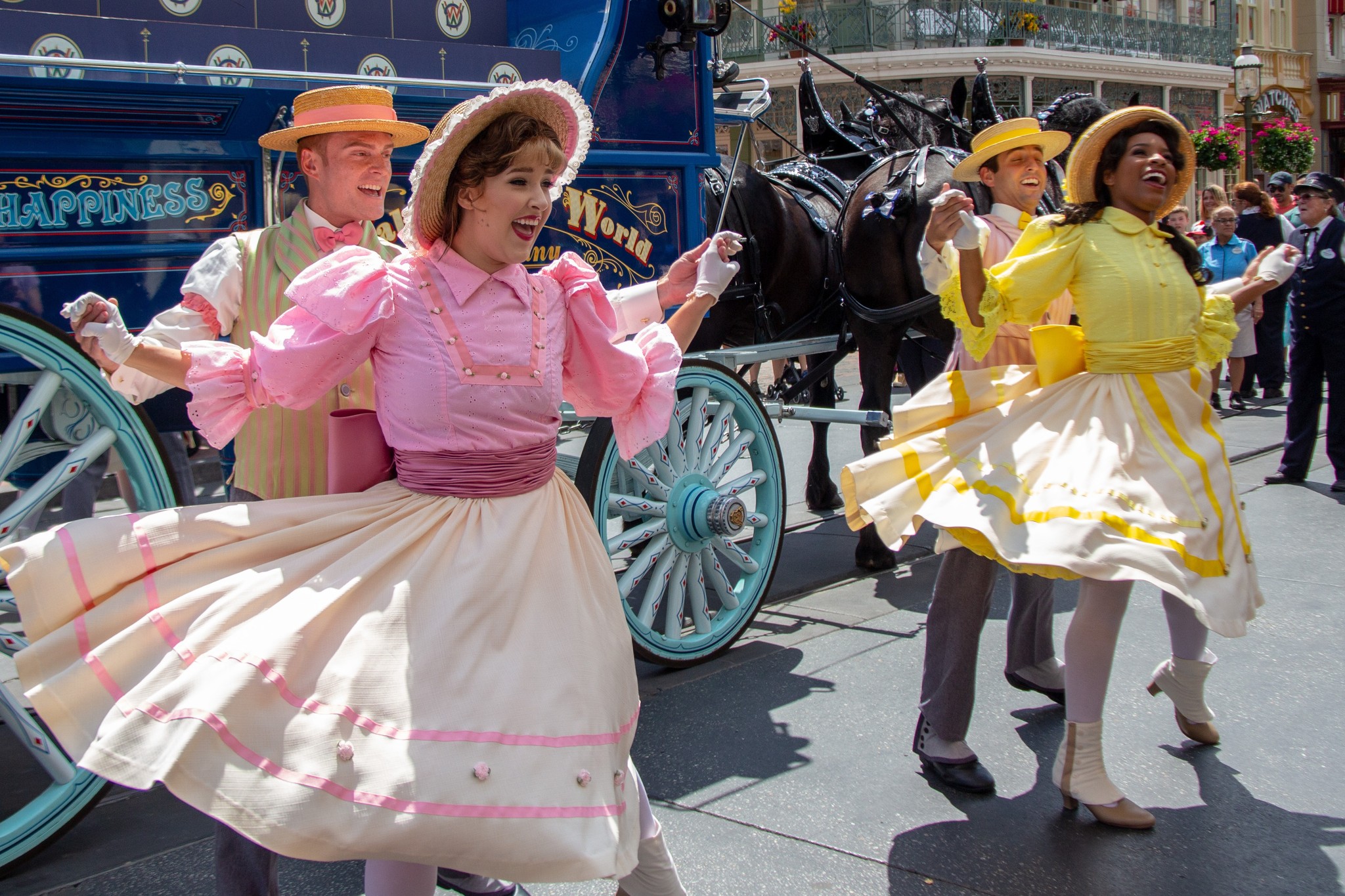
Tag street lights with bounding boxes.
[1233,42,1263,184]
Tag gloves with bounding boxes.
[60,291,145,365]
[686,231,742,307]
[930,188,981,251]
[1250,243,1302,290]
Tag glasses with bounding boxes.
[1269,186,1285,193]
[1215,217,1237,223]
[1230,199,1245,206]
[1291,193,1330,205]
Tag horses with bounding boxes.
[705,74,973,512]
[834,89,1144,569]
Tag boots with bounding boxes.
[1147,648,1222,745]
[1051,718,1157,830]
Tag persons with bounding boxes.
[1161,172,1345,411]
[915,117,1299,797]
[64,87,519,896]
[929,107,1303,829]
[61,77,742,896]
[1264,171,1345,492]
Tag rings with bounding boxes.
[1258,316,1260,317]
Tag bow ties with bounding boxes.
[313,221,364,253]
[1300,227,1320,235]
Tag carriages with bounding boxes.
[0,0,1149,875]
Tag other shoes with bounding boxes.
[919,744,996,792]
[1209,392,1221,409]
[1263,388,1284,398]
[801,370,809,377]
[1240,387,1257,398]
[713,61,740,88]
[1264,471,1304,484]
[1228,393,1246,410]
[1330,479,1345,490]
[436,874,532,896]
[1004,657,1065,706]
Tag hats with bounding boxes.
[1187,224,1213,238]
[1294,171,1345,204]
[1066,105,1196,220]
[401,78,593,258]
[1267,171,1293,187]
[258,84,431,153]
[952,117,1071,184]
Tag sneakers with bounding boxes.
[749,379,761,394]
[893,373,908,387]
[774,377,788,390]
